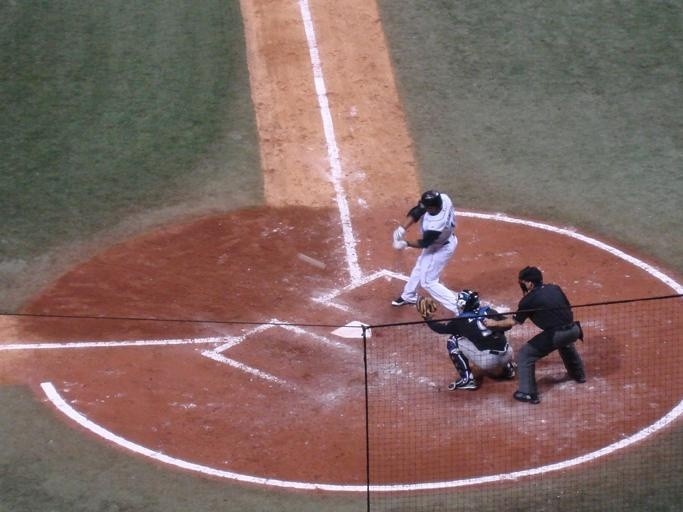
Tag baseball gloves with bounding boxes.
[416,295,436,320]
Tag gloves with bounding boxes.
[392,225,408,250]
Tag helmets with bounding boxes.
[421,190,442,206]
[456,288,480,315]
[518,266,543,297]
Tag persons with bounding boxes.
[389,189,463,317]
[483,264,586,405]
[414,286,515,391]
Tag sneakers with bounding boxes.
[448,378,479,390]
[564,369,586,383]
[506,359,518,378]
[392,297,410,307]
[513,390,539,404]
